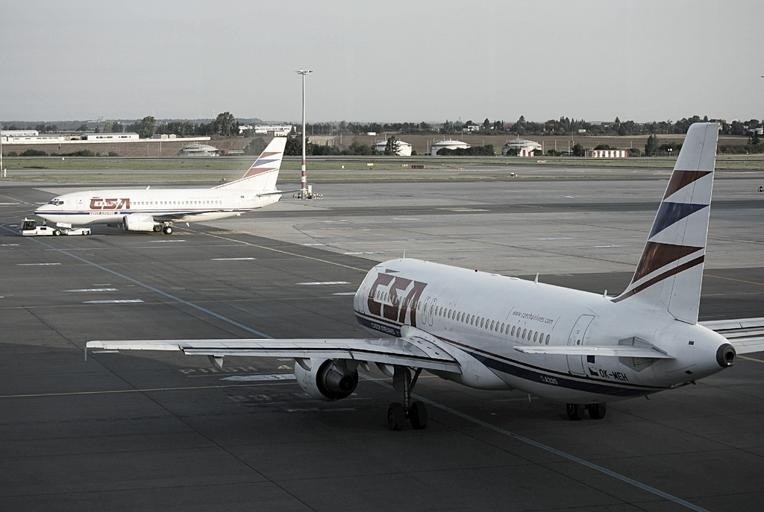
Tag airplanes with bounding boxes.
[32,135,310,237]
[82,120,764,432]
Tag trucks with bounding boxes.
[18,216,92,238]
